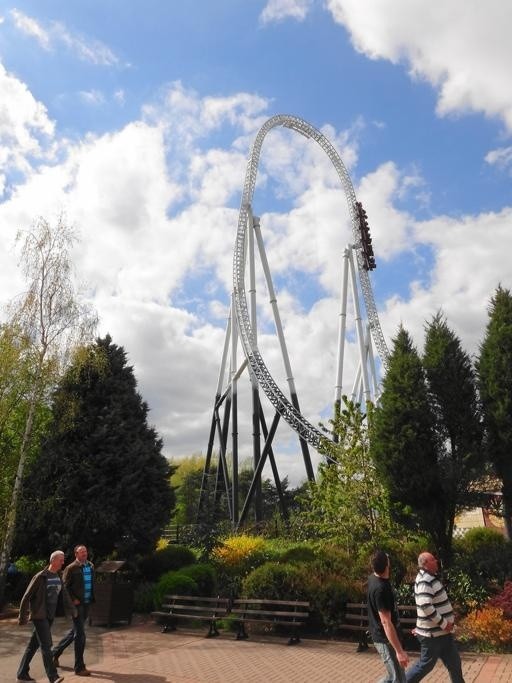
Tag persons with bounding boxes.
[367,551,409,683]
[16,550,78,683]
[405,552,464,683]
[51,545,96,676]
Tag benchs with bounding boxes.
[339,600,419,653]
[148,595,311,645]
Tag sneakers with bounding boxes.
[52,656,59,667]
[16,678,35,683]
[50,676,63,683]
[76,668,90,676]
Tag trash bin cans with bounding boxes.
[89,561,134,627]
